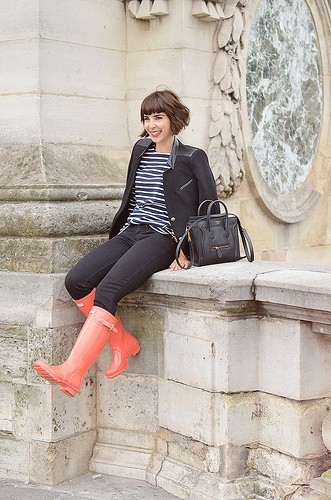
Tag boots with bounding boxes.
[32,305,118,397]
[72,287,140,380]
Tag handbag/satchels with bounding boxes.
[175,199,254,270]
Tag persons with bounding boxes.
[34,90,222,397]
